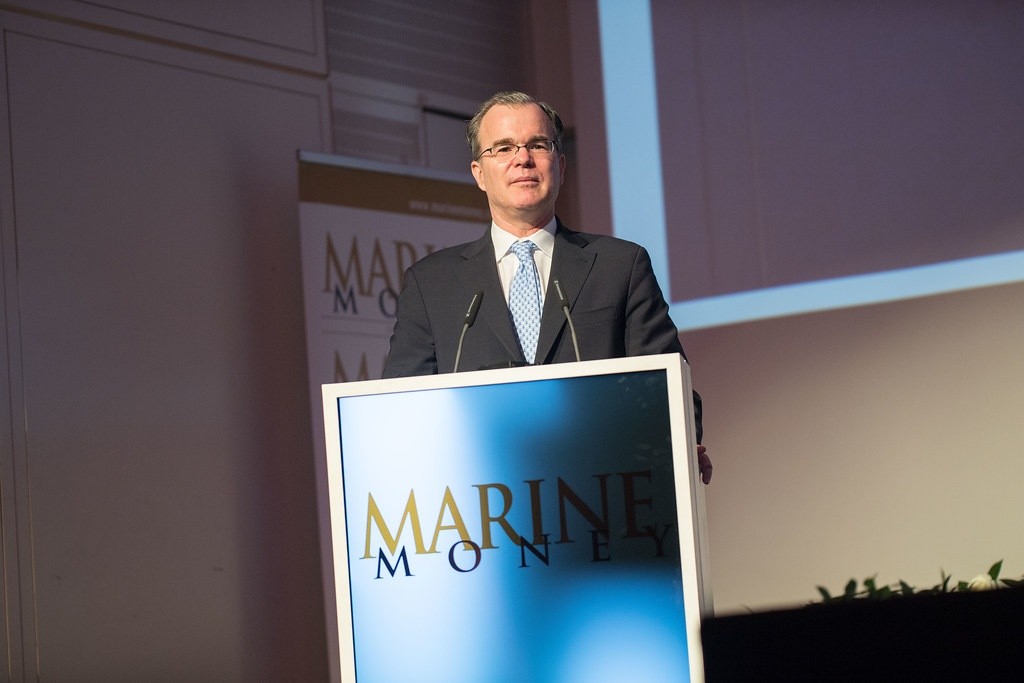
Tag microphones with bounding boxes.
[453,288,485,373]
[552,275,581,362]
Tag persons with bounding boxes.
[381,91,712,486]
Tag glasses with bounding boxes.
[475,139,559,162]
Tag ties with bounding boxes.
[507,241,544,365]
